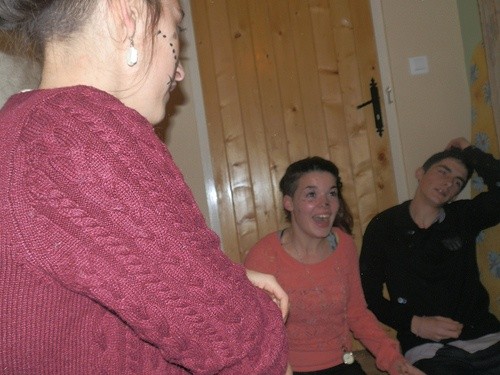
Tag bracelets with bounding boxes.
[417,316,426,338]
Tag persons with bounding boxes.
[243,156,427,374]
[0,0,293,375]
[360,137,500,375]
